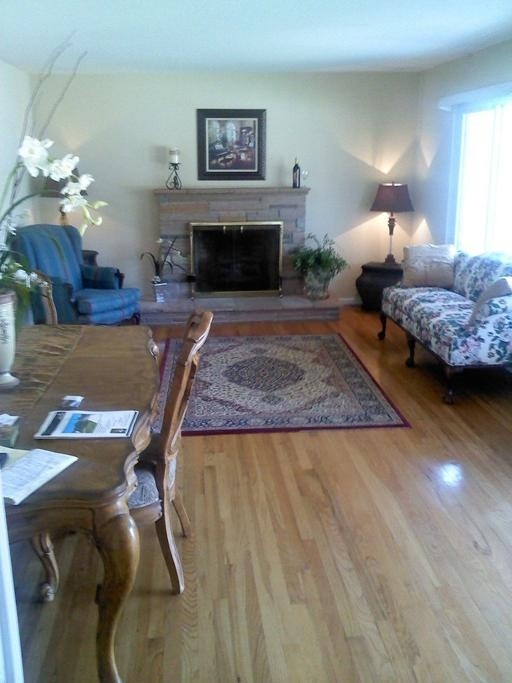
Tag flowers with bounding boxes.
[0,125,110,335]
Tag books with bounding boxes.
[36,408,140,441]
[0,445,78,505]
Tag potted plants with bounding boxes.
[291,233,348,300]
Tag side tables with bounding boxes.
[355,263,403,312]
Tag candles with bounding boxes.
[168,146,181,166]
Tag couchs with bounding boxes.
[377,251,512,406]
[7,225,140,327]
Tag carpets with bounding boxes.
[156,335,411,436]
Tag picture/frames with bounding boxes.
[196,109,266,180]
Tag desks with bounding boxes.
[0,309,160,683]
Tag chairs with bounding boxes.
[29,308,214,602]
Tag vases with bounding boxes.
[0,287,21,388]
[140,236,188,283]
[151,283,169,303]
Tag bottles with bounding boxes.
[293,157,300,188]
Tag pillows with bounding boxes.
[402,245,455,288]
[468,276,512,328]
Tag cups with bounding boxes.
[301,168,308,188]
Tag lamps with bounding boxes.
[370,181,414,265]
